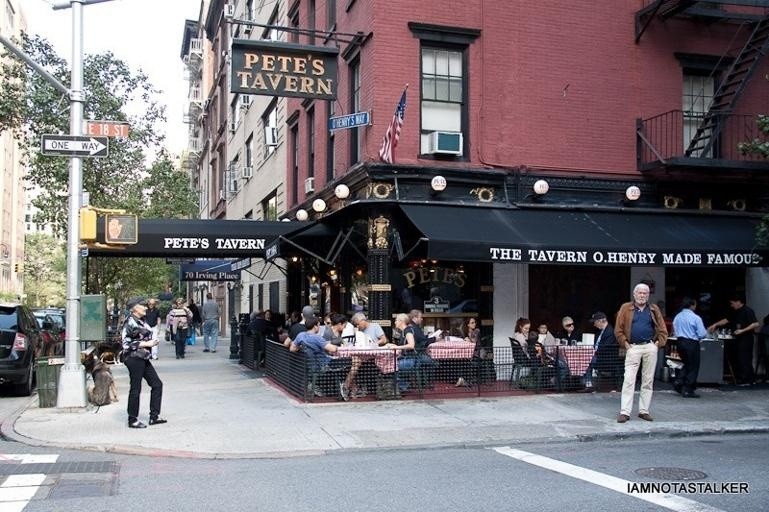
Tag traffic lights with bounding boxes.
[107,213,137,244]
[14,262,19,273]
[79,208,96,240]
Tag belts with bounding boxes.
[629,339,654,346]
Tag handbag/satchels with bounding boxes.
[165,330,170,342]
[397,357,420,371]
[376,374,401,401]
[186,325,192,337]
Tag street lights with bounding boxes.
[0,243,8,259]
[109,280,122,331]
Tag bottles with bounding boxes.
[670,343,680,358]
[662,365,670,384]
[707,328,731,341]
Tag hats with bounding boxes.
[588,312,607,323]
[175,298,187,305]
[126,296,147,309]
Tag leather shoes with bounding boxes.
[682,391,700,399]
[577,386,596,393]
[674,381,683,394]
[617,414,629,424]
[638,413,653,421]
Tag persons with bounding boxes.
[142,298,161,360]
[170,297,194,359]
[165,304,177,345]
[672,295,709,400]
[252,296,629,402]
[201,293,220,354]
[612,281,670,422]
[120,294,168,429]
[705,293,761,388]
[760,312,769,361]
[185,298,203,346]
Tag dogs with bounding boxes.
[87,353,119,414]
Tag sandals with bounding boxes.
[128,422,147,429]
[149,416,167,425]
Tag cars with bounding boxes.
[0,301,65,396]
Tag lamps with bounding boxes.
[625,185,641,201]
[296,209,309,222]
[304,176,315,194]
[281,217,292,223]
[431,176,447,192]
[533,178,550,196]
[334,183,350,201]
[312,198,326,213]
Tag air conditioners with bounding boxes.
[263,126,278,146]
[241,166,251,178]
[239,95,250,109]
[179,37,204,172]
[229,122,235,131]
[229,179,237,194]
[429,128,464,158]
[224,4,235,19]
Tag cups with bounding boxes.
[555,339,576,346]
[582,333,594,346]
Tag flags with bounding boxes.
[378,88,407,166]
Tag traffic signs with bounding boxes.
[41,134,110,156]
[81,119,128,139]
[329,111,371,130]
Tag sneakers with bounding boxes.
[340,382,350,401]
[308,383,324,397]
[454,377,471,387]
[152,355,158,360]
[175,354,185,359]
[203,348,216,352]
[351,390,368,399]
